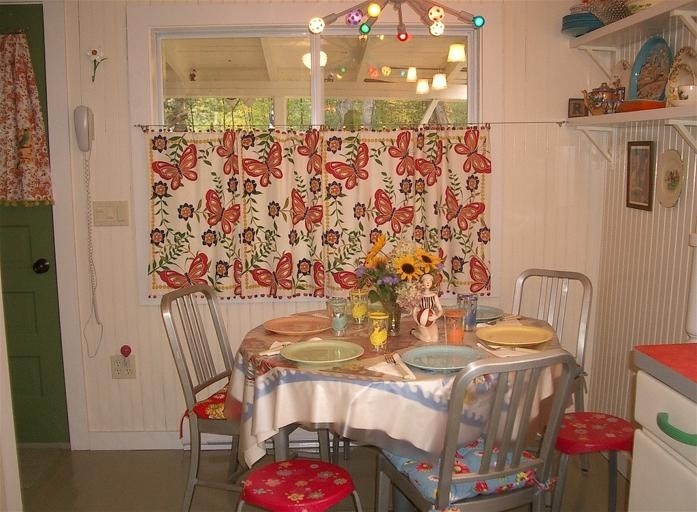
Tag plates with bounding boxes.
[669,100,697,106]
[402,346,488,370]
[628,37,671,102]
[622,99,666,111]
[443,303,502,320]
[657,148,683,207]
[561,9,604,37]
[475,326,553,347]
[280,339,365,366]
[264,317,333,335]
[609,59,631,97]
[664,47,697,106]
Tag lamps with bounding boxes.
[307,0,484,42]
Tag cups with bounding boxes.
[457,292,476,331]
[327,298,348,336]
[443,310,464,345]
[350,288,367,323]
[368,312,388,354]
[677,84,697,100]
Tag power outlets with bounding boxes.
[111,356,135,379]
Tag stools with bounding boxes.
[549,410,636,511]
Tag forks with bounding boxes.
[384,352,410,379]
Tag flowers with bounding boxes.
[85,45,109,83]
[355,235,443,328]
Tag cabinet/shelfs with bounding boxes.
[625,343,696,511]
[564,0,696,164]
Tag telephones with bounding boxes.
[74,105,95,152]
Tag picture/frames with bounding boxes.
[625,140,654,211]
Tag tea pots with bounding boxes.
[581,83,626,116]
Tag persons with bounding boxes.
[409,273,445,343]
